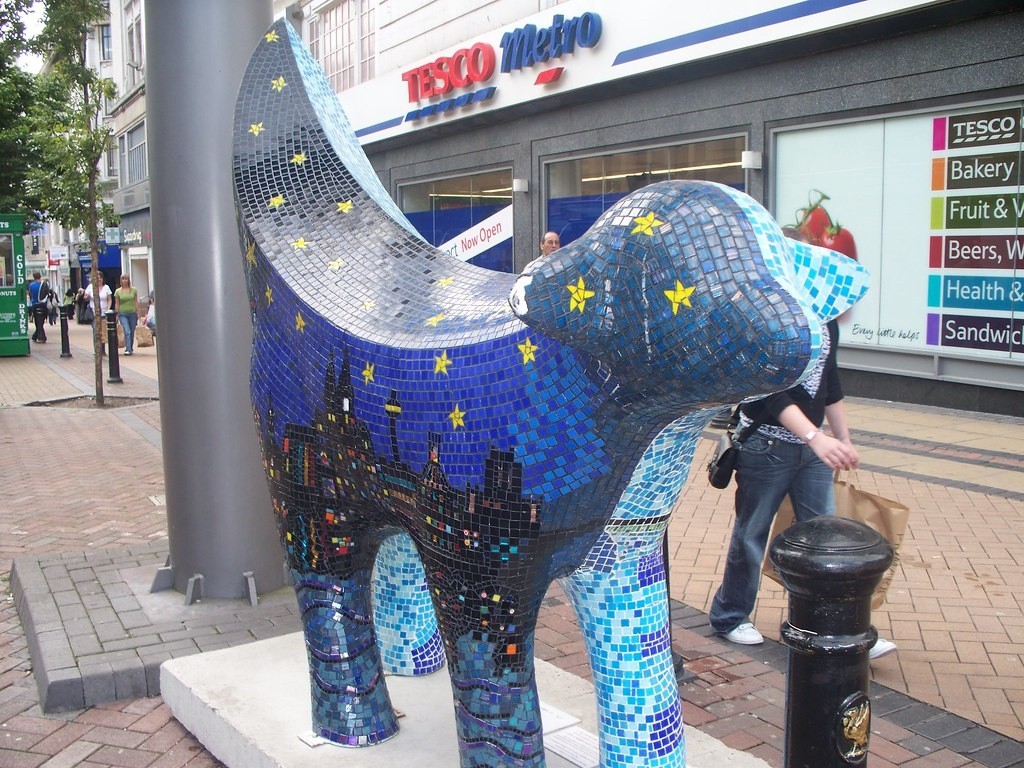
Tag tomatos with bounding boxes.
[799,207,856,261]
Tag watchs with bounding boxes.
[805,429,823,441]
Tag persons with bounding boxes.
[47,290,59,325]
[143,296,156,337]
[84,271,112,355]
[522,231,561,271]
[707,226,897,660]
[74,287,85,324]
[114,273,140,356]
[63,289,75,320]
[26,273,54,343]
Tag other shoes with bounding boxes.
[123,351,132,355]
[36,340,46,343]
[31,339,36,343]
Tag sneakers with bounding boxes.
[869,637,896,660]
[710,622,764,645]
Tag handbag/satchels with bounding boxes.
[116,315,126,347]
[706,431,742,490]
[136,319,155,347]
[762,464,909,611]
[78,300,94,325]
[52,294,58,307]
